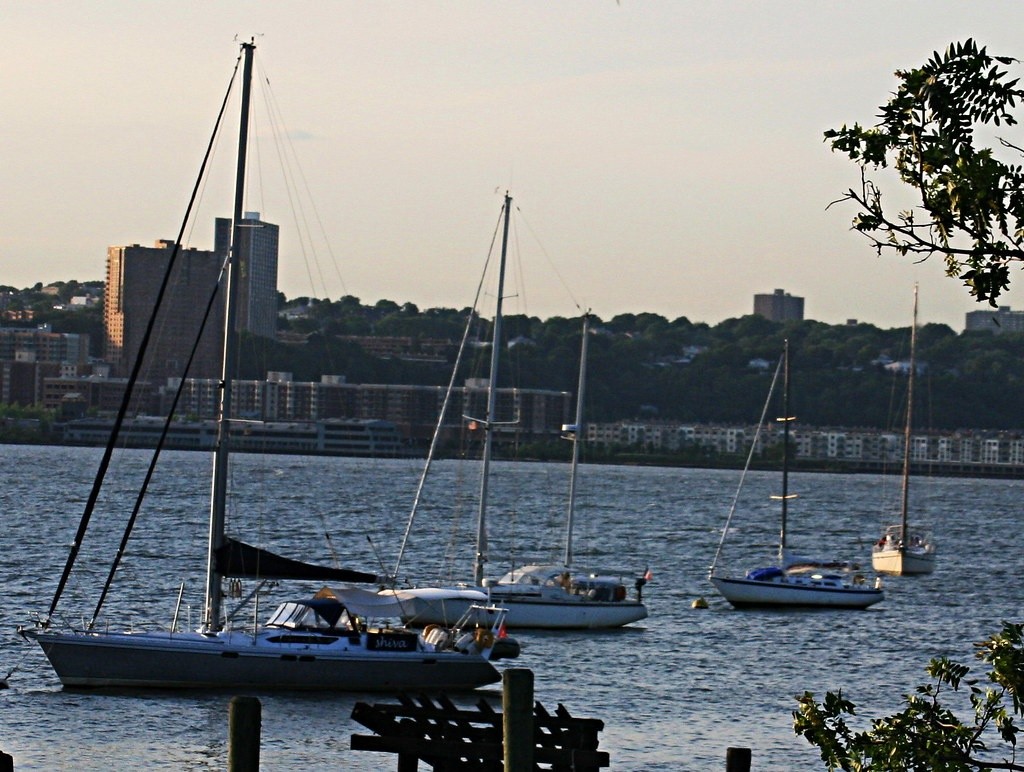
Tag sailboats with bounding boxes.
[706,338,886,610]
[17,38,506,702]
[870,279,938,578]
[380,190,650,634]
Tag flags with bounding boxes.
[644,567,652,580]
[492,622,500,635]
[498,620,507,638]
